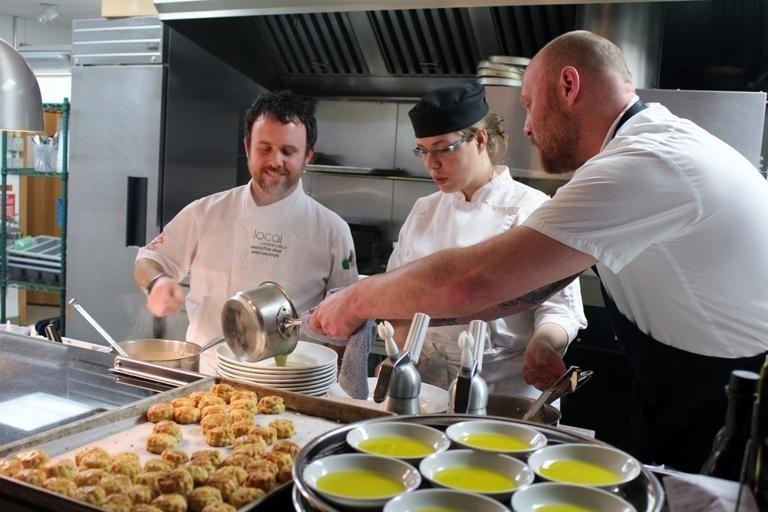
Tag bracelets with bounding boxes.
[144,272,172,299]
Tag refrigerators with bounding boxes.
[66,16,239,343]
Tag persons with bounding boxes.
[374,81,588,427]
[133,89,360,379]
[310,28,768,492]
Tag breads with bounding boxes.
[0,383,298,511]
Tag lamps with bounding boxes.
[0,39,44,132]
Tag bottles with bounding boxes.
[698,368,768,512]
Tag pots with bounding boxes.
[114,336,224,373]
[220,280,320,363]
[486,369,595,428]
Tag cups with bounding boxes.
[34,145,57,173]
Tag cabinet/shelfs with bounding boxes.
[0,97,70,336]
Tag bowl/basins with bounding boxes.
[527,443,642,486]
[510,488,636,512]
[347,421,451,458]
[302,453,421,502]
[418,448,535,494]
[445,419,547,453]
[382,488,510,512]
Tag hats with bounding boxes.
[407,83,490,138]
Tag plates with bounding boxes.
[327,377,450,415]
[216,340,339,398]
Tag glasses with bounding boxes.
[413,139,466,160]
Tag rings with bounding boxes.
[328,334,337,341]
